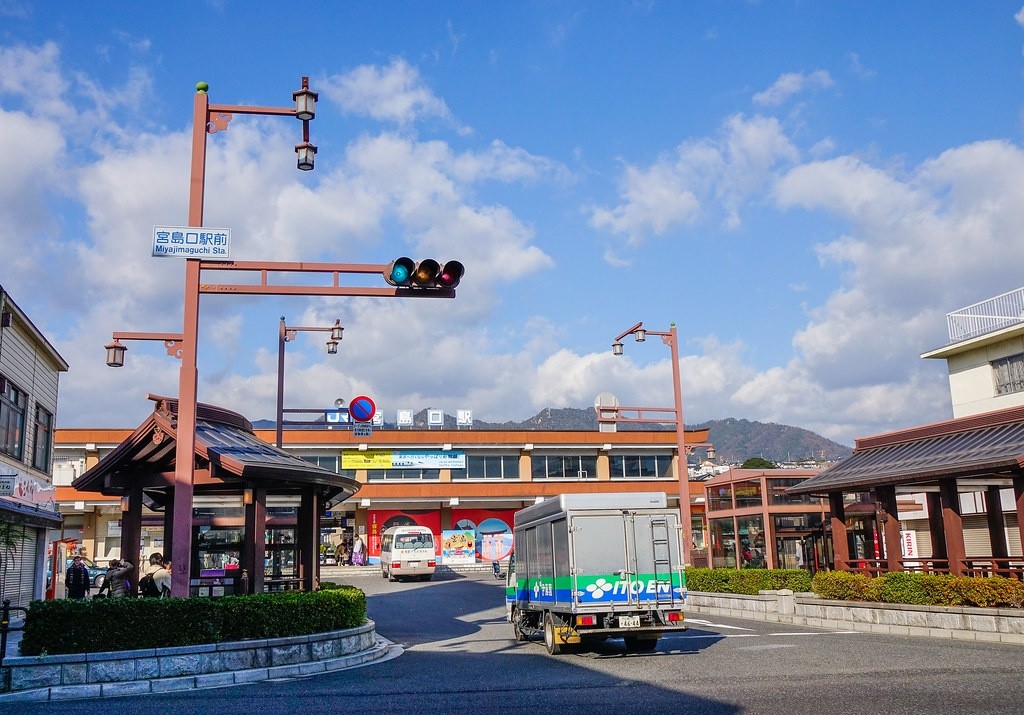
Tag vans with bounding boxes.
[320,551,337,566]
[381,525,435,582]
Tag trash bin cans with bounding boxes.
[492,560,501,578]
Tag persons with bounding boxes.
[211,553,239,569]
[65,556,90,600]
[283,535,292,566]
[335,539,353,566]
[744,549,752,569]
[146,553,171,599]
[795,541,804,569]
[99,559,134,598]
[352,534,364,566]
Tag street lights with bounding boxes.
[276,315,344,449]
[611,322,693,567]
[104,76,318,594]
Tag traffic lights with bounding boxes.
[383,256,465,289]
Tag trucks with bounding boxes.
[491,490,690,656]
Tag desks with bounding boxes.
[265,579,304,591]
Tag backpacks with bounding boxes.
[138,568,162,598]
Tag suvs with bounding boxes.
[47,556,109,588]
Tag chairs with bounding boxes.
[388,541,432,550]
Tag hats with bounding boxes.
[110,559,121,569]
